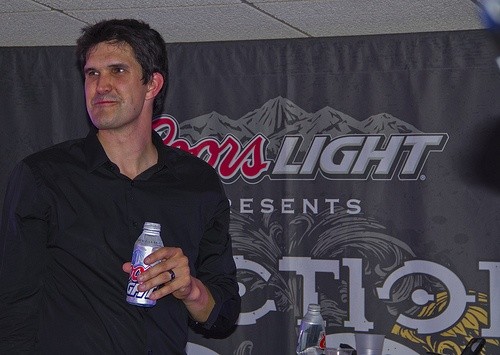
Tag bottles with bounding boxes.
[296,304,326,355]
[127,222,164,306]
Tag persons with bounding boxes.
[0,19,240,355]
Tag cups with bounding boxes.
[355,333,386,355]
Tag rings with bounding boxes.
[168,269,175,280]
